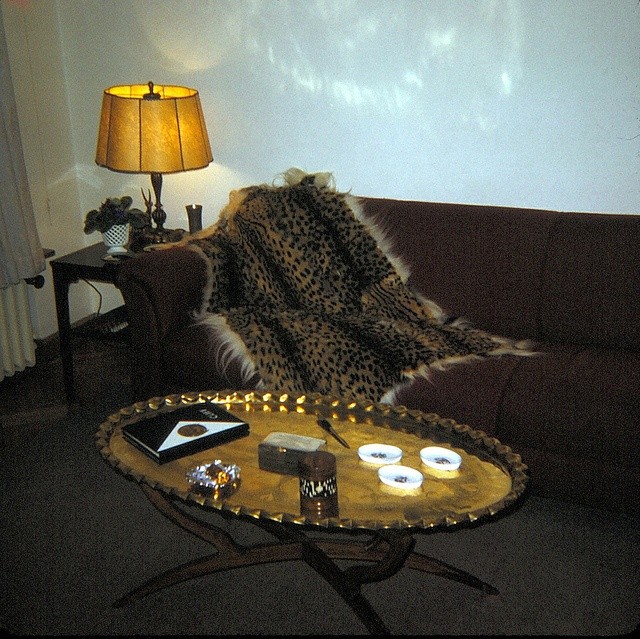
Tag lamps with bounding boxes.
[94,81,217,256]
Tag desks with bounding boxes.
[50,229,204,403]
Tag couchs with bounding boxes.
[114,184,637,516]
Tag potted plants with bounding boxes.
[83,196,146,266]
